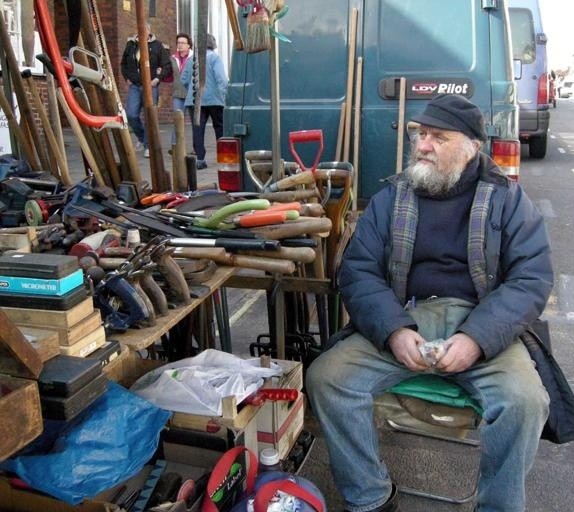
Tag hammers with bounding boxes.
[70,227,315,273]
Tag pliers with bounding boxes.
[139,191,192,213]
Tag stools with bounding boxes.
[378,375,485,505]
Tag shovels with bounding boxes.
[287,129,332,361]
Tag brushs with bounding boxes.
[245,0,272,54]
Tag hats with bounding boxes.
[410,93,488,142]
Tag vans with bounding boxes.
[507,0,556,159]
[215,0,522,214]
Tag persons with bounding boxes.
[180,33,227,169]
[156,33,197,156]
[305,95,574,512]
[121,22,171,158]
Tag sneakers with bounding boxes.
[135,141,150,158]
[197,160,207,169]
[368,482,399,512]
